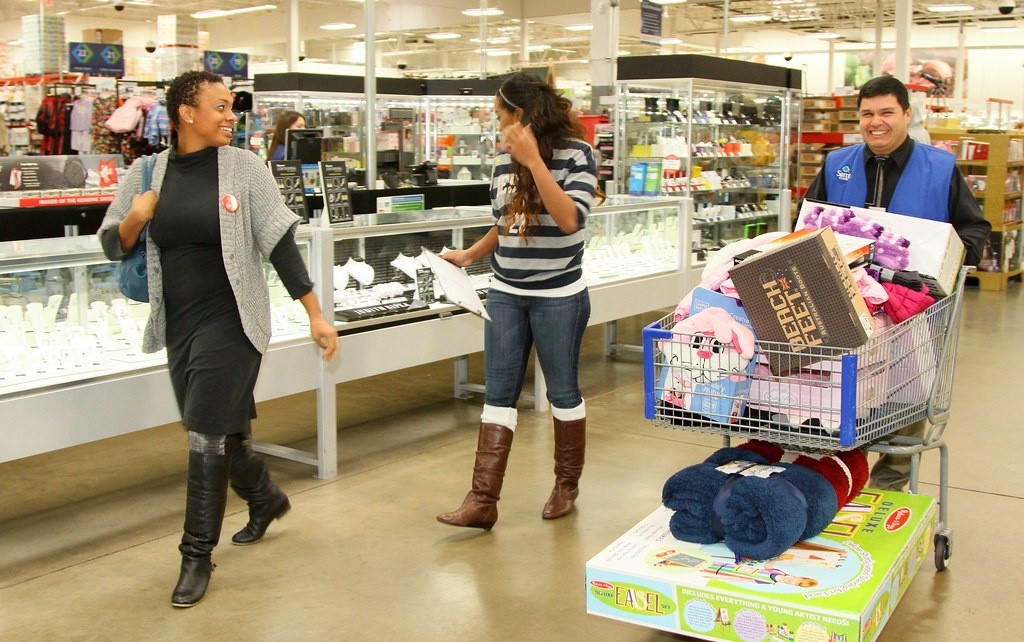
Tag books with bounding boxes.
[932,136,1024,273]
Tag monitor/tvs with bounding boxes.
[284,129,324,165]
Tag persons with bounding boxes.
[97,69,340,610]
[265,110,308,169]
[435,70,607,533]
[790,73,994,494]
[907,91,931,146]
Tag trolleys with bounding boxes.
[639,255,974,574]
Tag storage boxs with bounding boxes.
[746,321,893,418]
[728,224,877,377]
[794,198,969,298]
[886,310,937,406]
[655,287,759,427]
[799,96,862,188]
[583,484,938,642]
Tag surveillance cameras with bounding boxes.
[144,40,156,53]
[998,0,1016,14]
[397,59,408,70]
[784,53,792,61]
[299,53,306,61]
[114,1,124,11]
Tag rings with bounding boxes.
[506,145,512,153]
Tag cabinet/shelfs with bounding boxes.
[252,73,509,191]
[929,126,1024,292]
[616,55,803,270]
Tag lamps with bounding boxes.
[563,23,595,30]
[461,8,503,16]
[728,12,772,23]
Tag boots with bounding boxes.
[171,449,230,608]
[228,420,292,546]
[542,415,587,519]
[436,422,513,531]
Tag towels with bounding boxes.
[661,438,869,560]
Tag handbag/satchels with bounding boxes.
[119,153,158,303]
